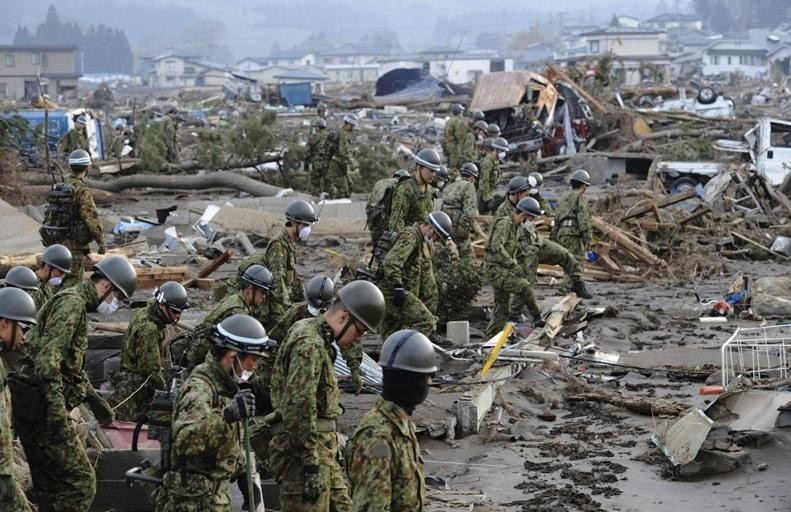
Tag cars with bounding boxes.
[632,86,735,120]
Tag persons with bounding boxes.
[299,118,333,200]
[69,115,91,159]
[107,124,135,160]
[443,103,509,214]
[310,101,330,135]
[367,148,599,347]
[50,150,106,293]
[163,106,187,163]
[265,278,386,512]
[261,197,304,305]
[324,111,359,200]
[158,313,284,512]
[185,263,277,369]
[258,271,365,397]
[345,325,439,512]
[1,243,191,511]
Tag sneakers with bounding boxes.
[533,311,546,327]
[570,281,593,299]
[428,330,453,346]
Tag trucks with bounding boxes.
[655,114,791,196]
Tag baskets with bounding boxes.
[721,324,791,393]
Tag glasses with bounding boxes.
[172,309,181,314]
[306,288,337,306]
[115,288,125,300]
[16,320,33,336]
[257,289,265,295]
[27,289,35,297]
[428,372,435,379]
[349,313,369,335]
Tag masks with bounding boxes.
[96,291,120,316]
[46,272,62,290]
[424,232,435,250]
[230,352,255,384]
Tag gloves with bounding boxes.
[302,465,322,504]
[393,284,404,307]
[351,368,362,396]
[90,395,115,428]
[224,388,257,424]
[96,238,105,255]
[237,472,260,512]
[588,251,600,262]
[508,263,526,278]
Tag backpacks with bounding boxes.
[169,295,250,369]
[323,130,340,160]
[39,172,82,248]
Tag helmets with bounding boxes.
[304,275,387,335]
[377,329,438,374]
[0,243,138,326]
[241,264,278,294]
[284,198,318,226]
[451,103,509,152]
[67,107,135,166]
[155,281,191,312]
[392,148,591,244]
[310,102,359,127]
[206,314,278,359]
